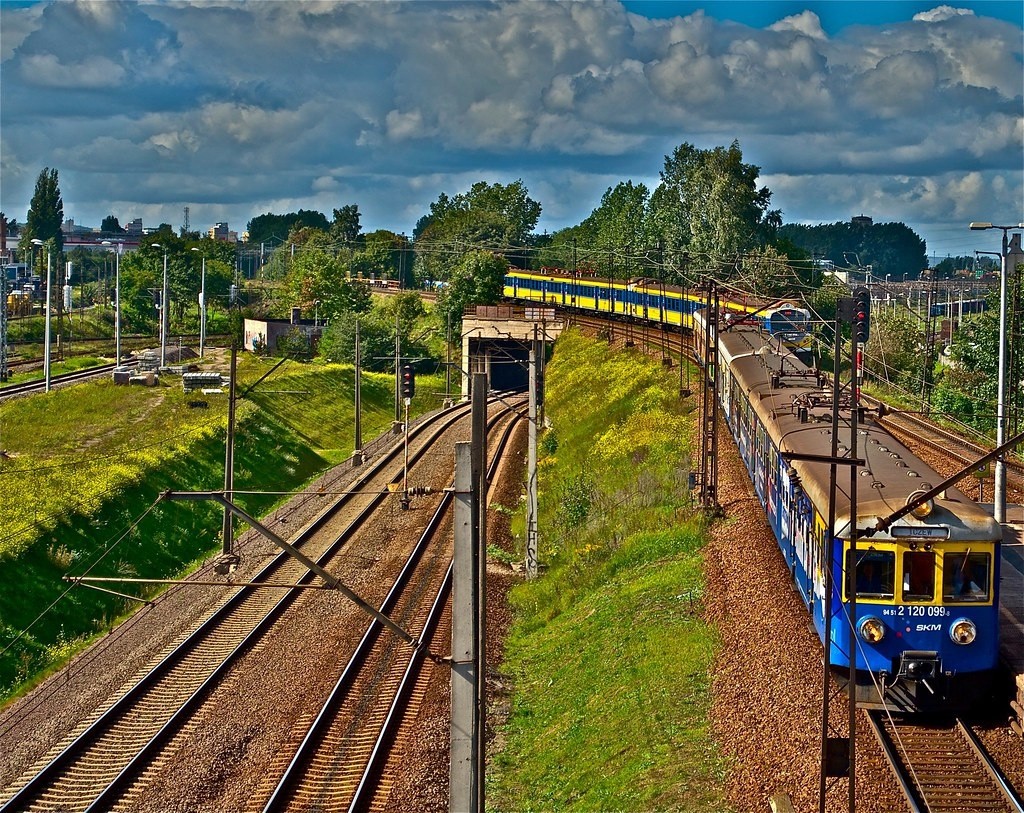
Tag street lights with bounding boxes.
[969,220,1024,524]
[190,247,205,360]
[150,243,168,367]
[100,240,120,368]
[31,237,52,393]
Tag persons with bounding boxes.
[946,563,986,598]
[847,556,884,597]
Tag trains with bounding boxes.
[423,266,815,358]
[688,304,1005,682]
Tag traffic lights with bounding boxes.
[400,362,415,398]
[853,287,870,343]
